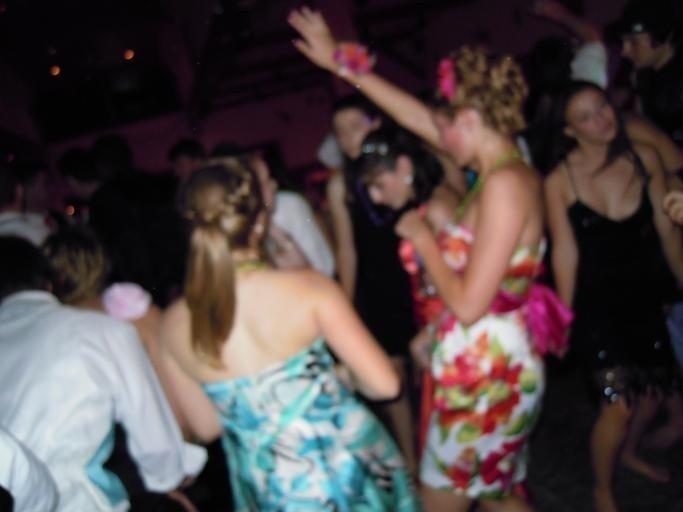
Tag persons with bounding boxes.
[0,2,683,512]
